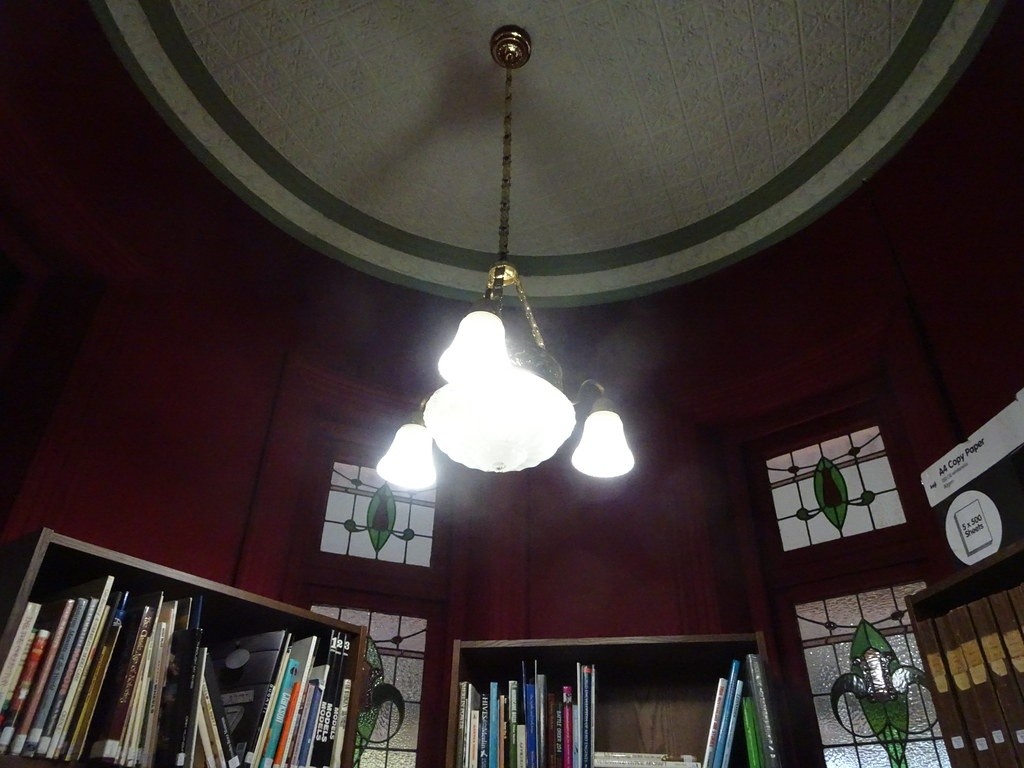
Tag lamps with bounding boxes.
[375,25,636,491]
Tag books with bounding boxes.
[917,585,1024,768]
[0,576,352,768]
[454,654,779,768]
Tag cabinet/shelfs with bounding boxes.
[0,528,368,768]
[904,538,1024,768]
[446,631,770,768]
